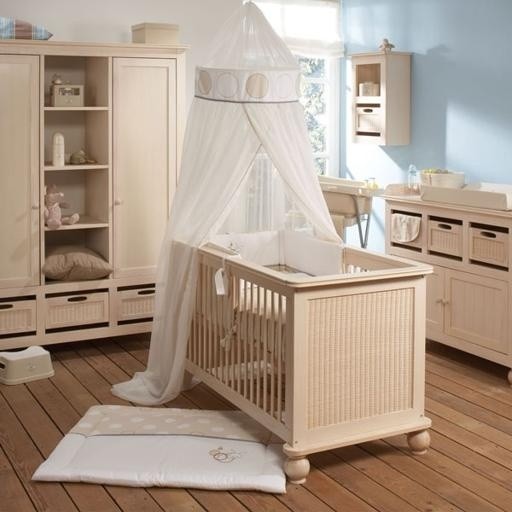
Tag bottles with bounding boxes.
[51,132,65,168]
[407,164,421,200]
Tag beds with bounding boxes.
[167,229,434,486]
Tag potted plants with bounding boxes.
[419,168,455,188]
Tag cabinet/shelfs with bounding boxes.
[0,37,194,357]
[348,54,410,148]
[376,182,511,385]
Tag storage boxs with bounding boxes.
[131,22,180,45]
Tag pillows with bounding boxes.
[0,16,53,41]
[41,244,111,281]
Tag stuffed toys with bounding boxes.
[43,183,80,230]
